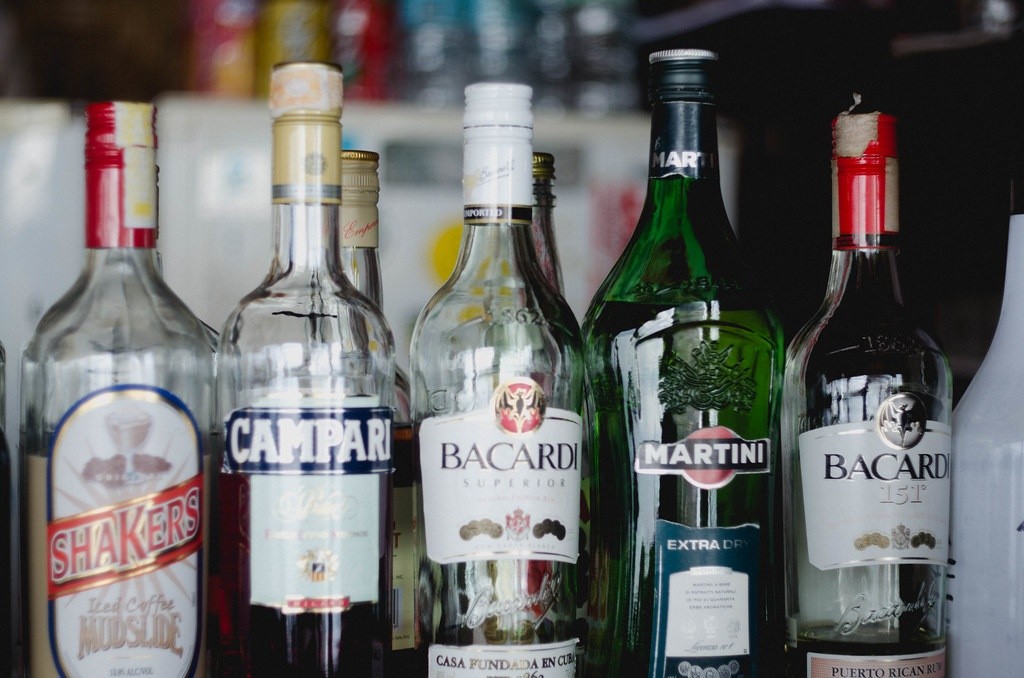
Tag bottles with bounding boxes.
[410,50,957,678]
[211,55,398,678]
[332,148,425,678]
[17,93,223,678]
